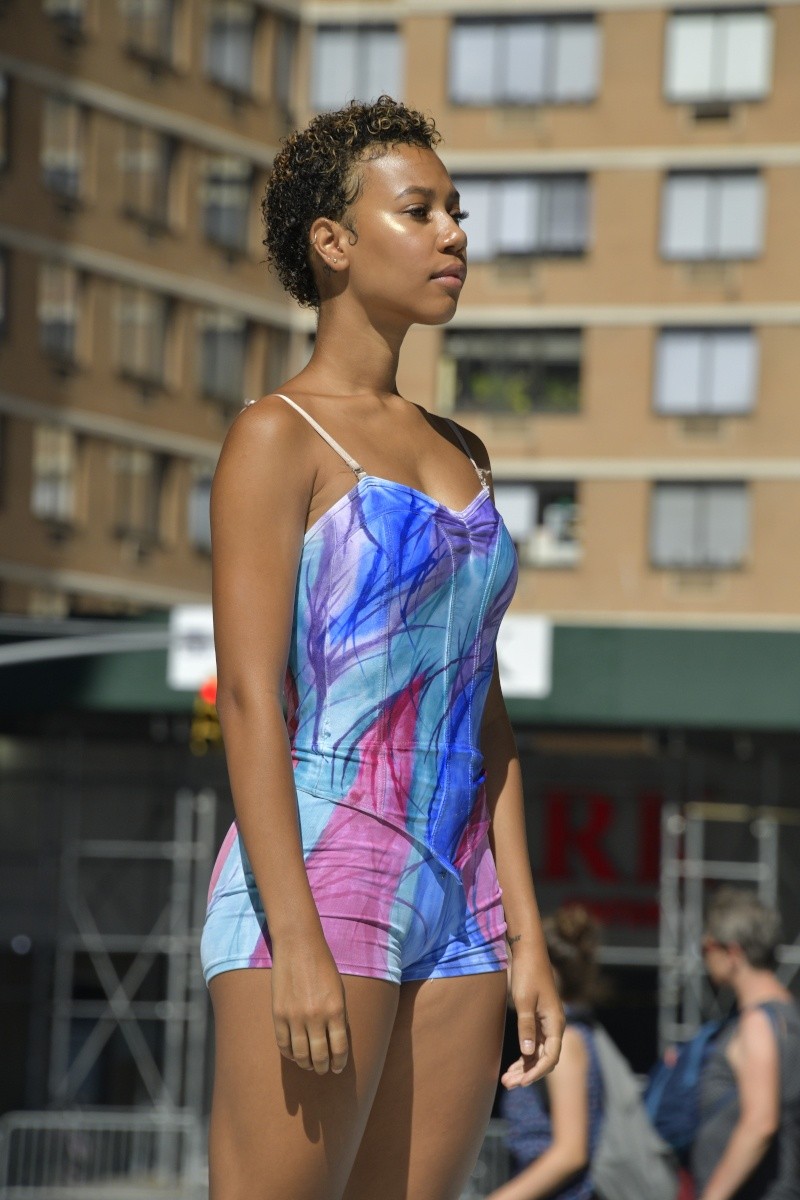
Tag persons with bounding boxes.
[460,905,616,1199]
[199,91,566,1200]
[681,887,800,1200]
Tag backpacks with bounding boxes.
[644,1000,775,1170]
[589,1024,681,1200]
